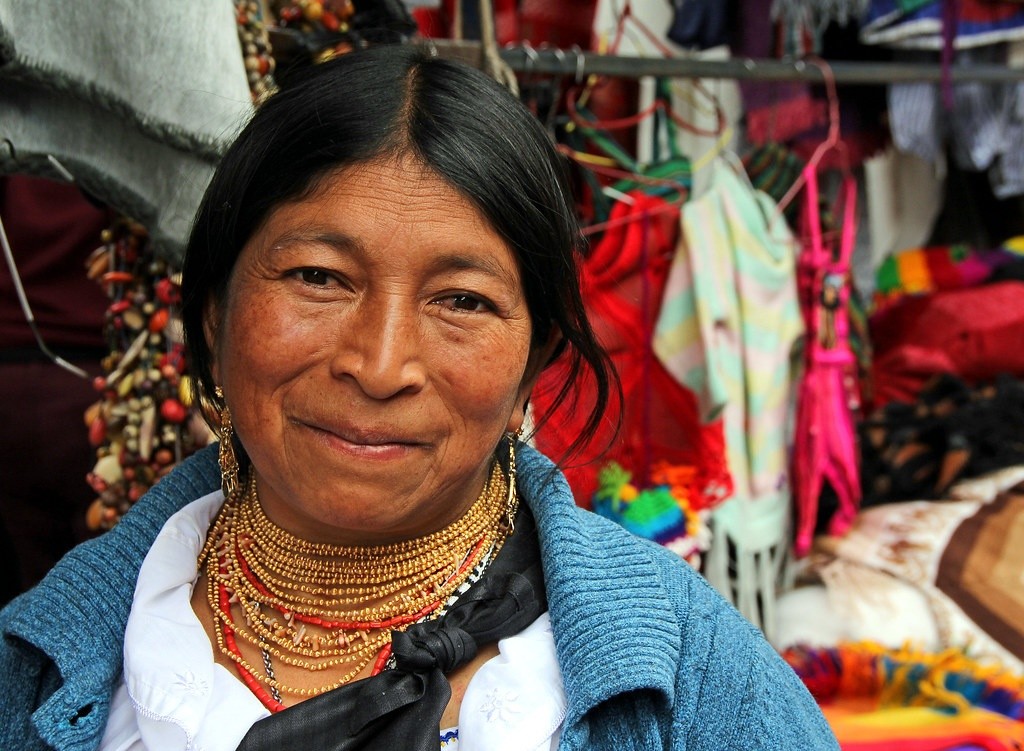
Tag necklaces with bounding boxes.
[198,463,506,713]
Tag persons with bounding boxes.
[1,44,838,750]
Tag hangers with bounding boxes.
[468,0,865,245]
